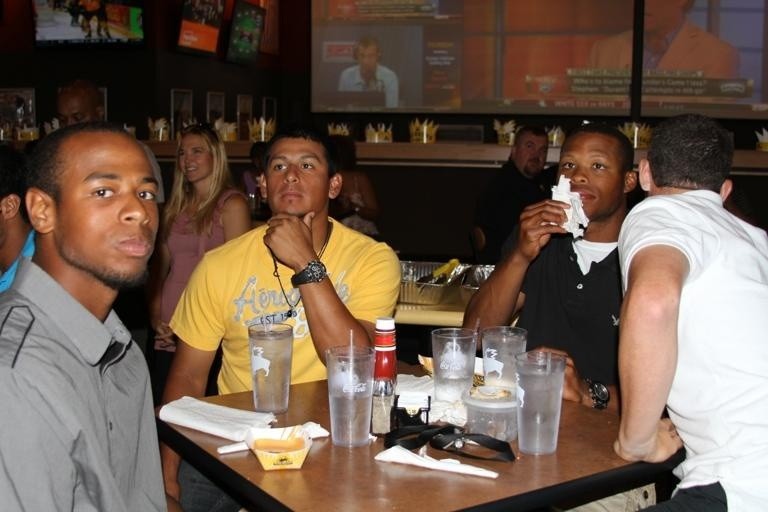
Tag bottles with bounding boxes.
[371,316,397,435]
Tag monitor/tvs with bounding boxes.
[305,0,638,142]
[175,0,226,57]
[639,0,768,129]
[224,0,266,66]
[31,0,146,48]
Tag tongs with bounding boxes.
[417,257,460,287]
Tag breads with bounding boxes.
[254,437,305,453]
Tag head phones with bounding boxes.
[352,40,382,62]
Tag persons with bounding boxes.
[338,33,400,107]
[587,1,741,79]
[1,143,36,296]
[81,1,115,47]
[0,123,166,512]
[148,121,253,352]
[460,123,638,416]
[480,125,556,244]
[54,82,105,125]
[614,111,768,511]
[160,128,402,512]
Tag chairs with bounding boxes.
[467,224,487,264]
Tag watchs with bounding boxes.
[588,374,611,410]
[291,261,327,288]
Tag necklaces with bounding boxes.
[272,222,331,318]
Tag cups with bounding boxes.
[514,348,568,458]
[247,319,292,414]
[459,388,522,440]
[482,325,529,381]
[431,326,478,400]
[325,345,375,449]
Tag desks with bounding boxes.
[392,278,519,332]
[155,362,685,512]
